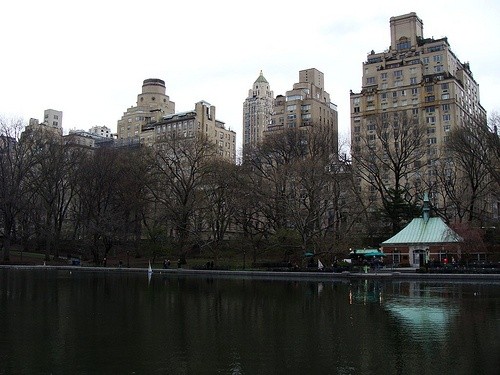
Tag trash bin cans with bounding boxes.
[71,260,80,266]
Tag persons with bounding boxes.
[162,259,170,268]
